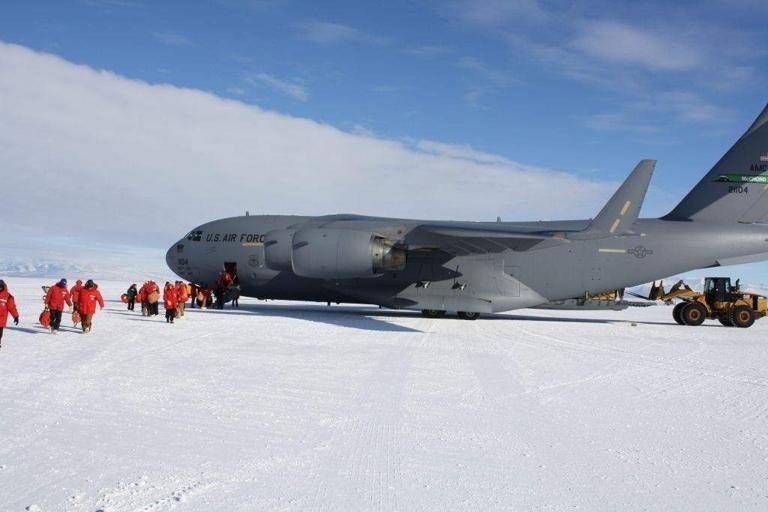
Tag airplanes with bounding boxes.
[165,104,768,323]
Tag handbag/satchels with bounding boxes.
[72,311,81,323]
[136,294,142,302]
[147,291,161,304]
[39,310,51,328]
[121,294,129,303]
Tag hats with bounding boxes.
[59,279,94,288]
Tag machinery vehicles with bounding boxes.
[646,277,768,328]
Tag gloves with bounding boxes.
[14,317,19,325]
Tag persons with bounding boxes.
[77,279,104,334]
[69,279,84,314]
[0,278,19,351]
[43,277,73,334]
[126,269,239,324]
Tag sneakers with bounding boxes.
[201,306,208,309]
[190,306,195,308]
